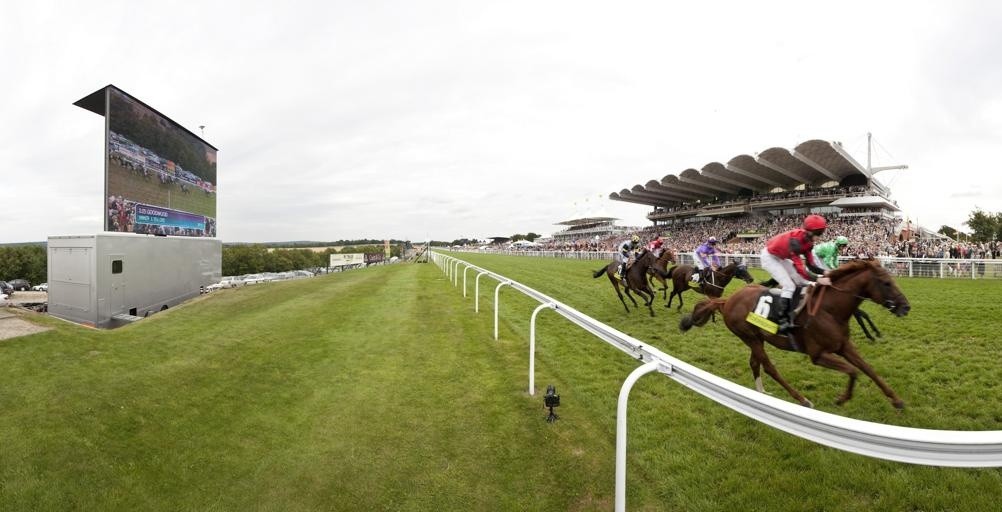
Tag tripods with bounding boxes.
[546,407,560,422]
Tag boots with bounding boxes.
[776,294,799,333]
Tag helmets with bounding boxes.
[708,236,716,246]
[630,234,640,243]
[833,235,848,247]
[657,238,666,244]
[802,213,831,231]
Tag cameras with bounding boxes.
[544,385,560,407]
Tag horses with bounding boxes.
[678,251,911,410]
[654,257,754,323]
[649,247,677,301]
[759,252,882,342]
[593,248,659,318]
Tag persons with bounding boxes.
[646,182,899,280]
[616,234,644,287]
[109,130,217,239]
[759,213,833,329]
[508,228,646,258]
[692,236,723,286]
[900,233,1001,277]
